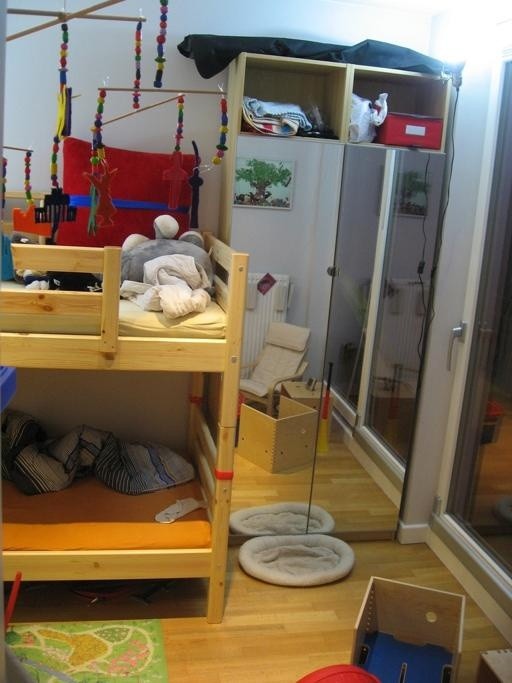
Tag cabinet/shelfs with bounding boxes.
[206,50,454,554]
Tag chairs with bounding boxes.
[241,320,312,417]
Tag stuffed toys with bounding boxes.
[118,210,218,297]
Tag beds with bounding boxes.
[0,226,250,624]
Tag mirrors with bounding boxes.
[234,131,446,535]
[234,152,295,211]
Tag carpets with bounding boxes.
[2,617,167,683]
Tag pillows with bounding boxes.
[56,136,201,248]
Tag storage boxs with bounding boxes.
[378,110,442,149]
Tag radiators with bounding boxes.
[241,273,294,381]
